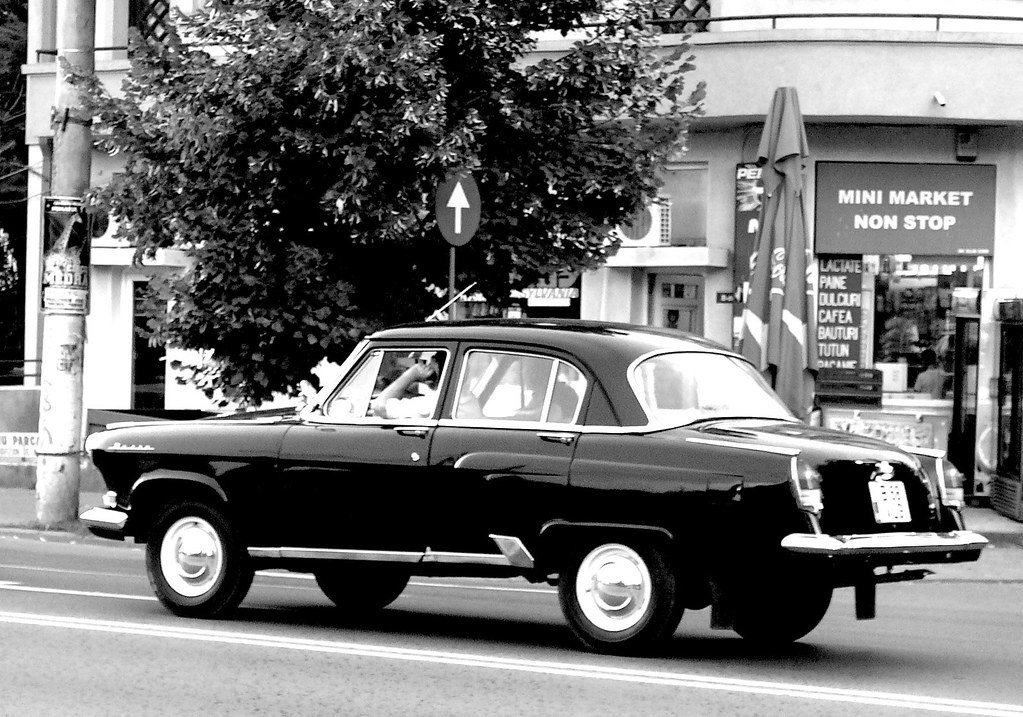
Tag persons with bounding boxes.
[665,308,681,329]
[914,347,945,398]
[942,333,955,400]
[372,350,482,422]
[495,354,579,424]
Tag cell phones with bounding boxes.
[426,373,438,382]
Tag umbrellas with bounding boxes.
[733,88,819,425]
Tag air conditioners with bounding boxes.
[614,194,672,248]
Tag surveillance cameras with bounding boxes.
[934,92,946,106]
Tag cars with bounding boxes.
[78,314,990,661]
